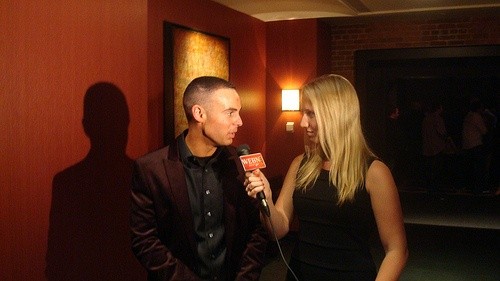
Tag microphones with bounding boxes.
[483,108,497,119]
[233,144,271,218]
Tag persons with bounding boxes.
[129,76,273,281]
[243,74,409,281]
[380,96,497,194]
